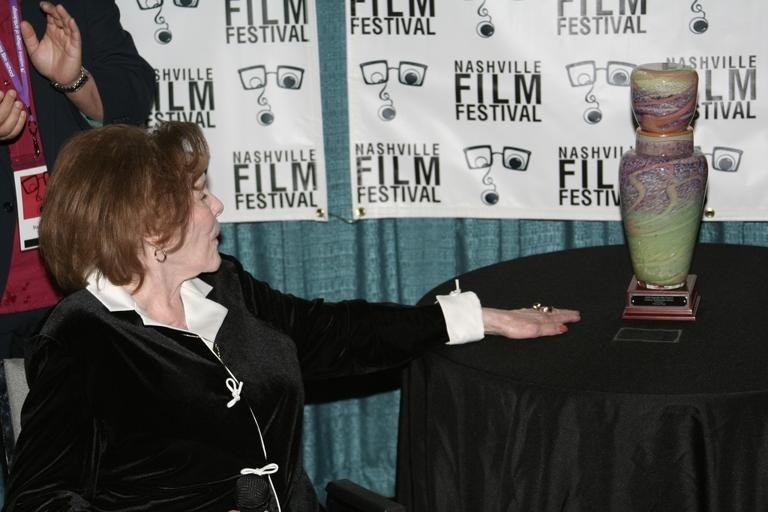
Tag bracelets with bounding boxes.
[50,65,88,93]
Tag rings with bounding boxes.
[532,302,542,311]
[543,305,553,313]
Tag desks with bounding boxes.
[395,242,766,511]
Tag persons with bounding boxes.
[0,0,141,403]
[3,121,583,512]
